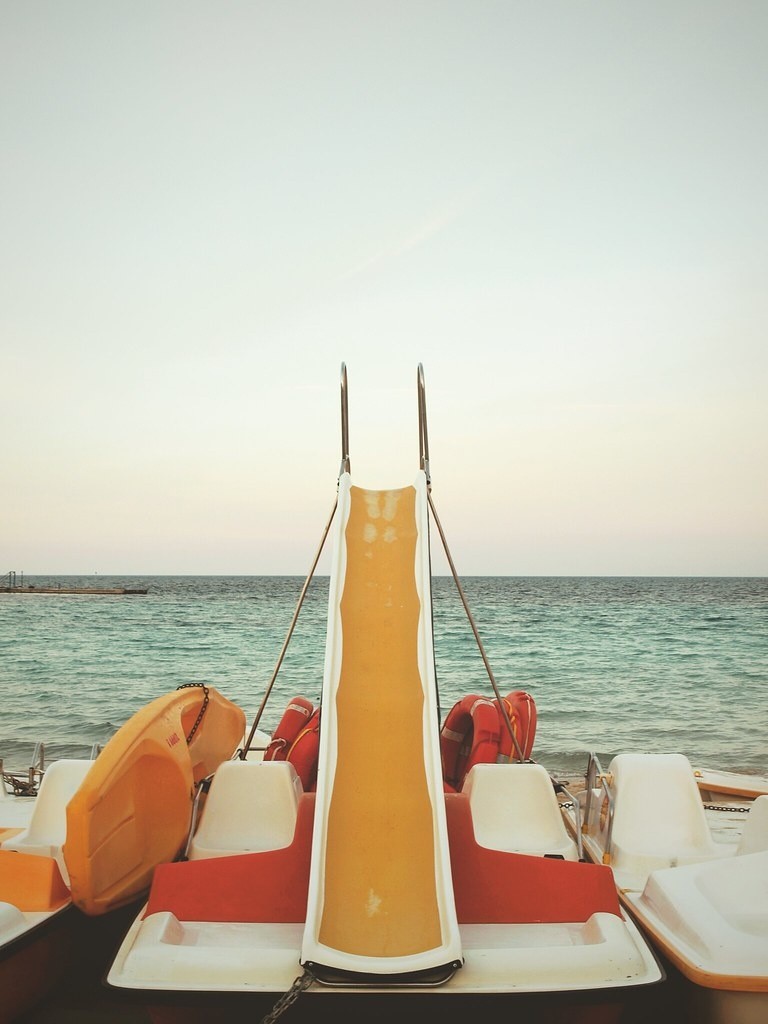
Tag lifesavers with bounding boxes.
[261,694,321,792]
[439,689,539,793]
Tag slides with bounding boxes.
[297,466,465,988]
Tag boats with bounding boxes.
[0,571,148,595]
[102,361,668,1024]
[554,751,767,992]
[0,756,98,952]
[63,686,246,918]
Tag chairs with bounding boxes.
[1,759,95,885]
[575,752,715,875]
[461,763,581,863]
[189,759,305,860]
